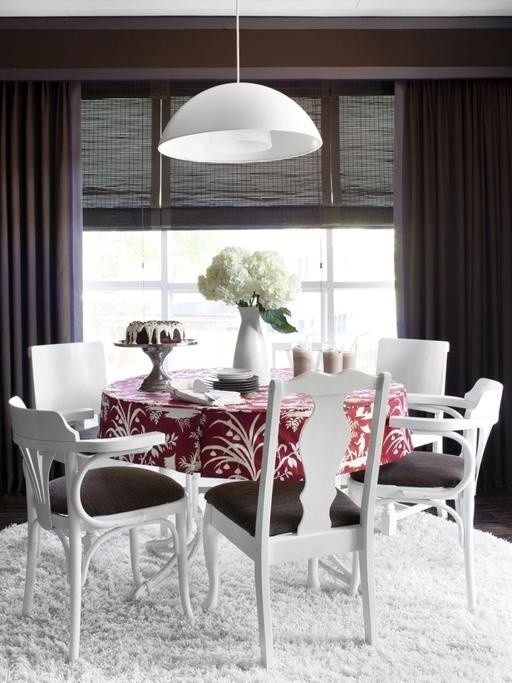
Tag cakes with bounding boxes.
[125,321,186,344]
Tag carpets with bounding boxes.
[0,480,512,681]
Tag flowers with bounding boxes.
[198,245,301,334]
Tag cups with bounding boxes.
[343,352,356,370]
[323,349,342,374]
[293,348,312,377]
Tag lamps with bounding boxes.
[157,1,324,164]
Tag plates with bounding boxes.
[204,368,259,393]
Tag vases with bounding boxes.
[233,306,270,386]
